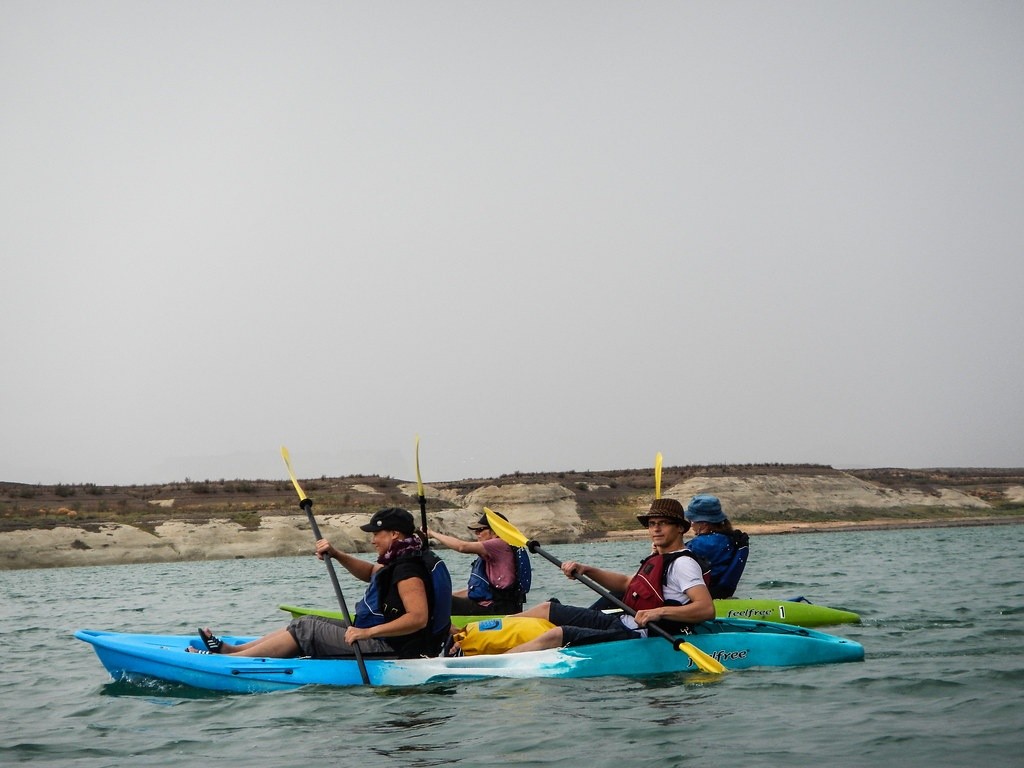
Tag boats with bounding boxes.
[74,618,867,693]
[281,598,863,628]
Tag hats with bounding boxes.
[359,507,415,535]
[684,495,727,522]
[467,512,509,530]
[636,498,691,534]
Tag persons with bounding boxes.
[448,498,717,655]
[587,494,736,610]
[185,508,448,659]
[420,512,526,617]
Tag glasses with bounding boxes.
[477,528,488,532]
[647,521,679,528]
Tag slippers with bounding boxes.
[198,628,224,652]
[185,648,214,654]
[444,634,465,658]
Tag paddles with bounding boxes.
[279,445,369,685]
[653,453,664,499]
[413,433,428,532]
[481,505,726,674]
